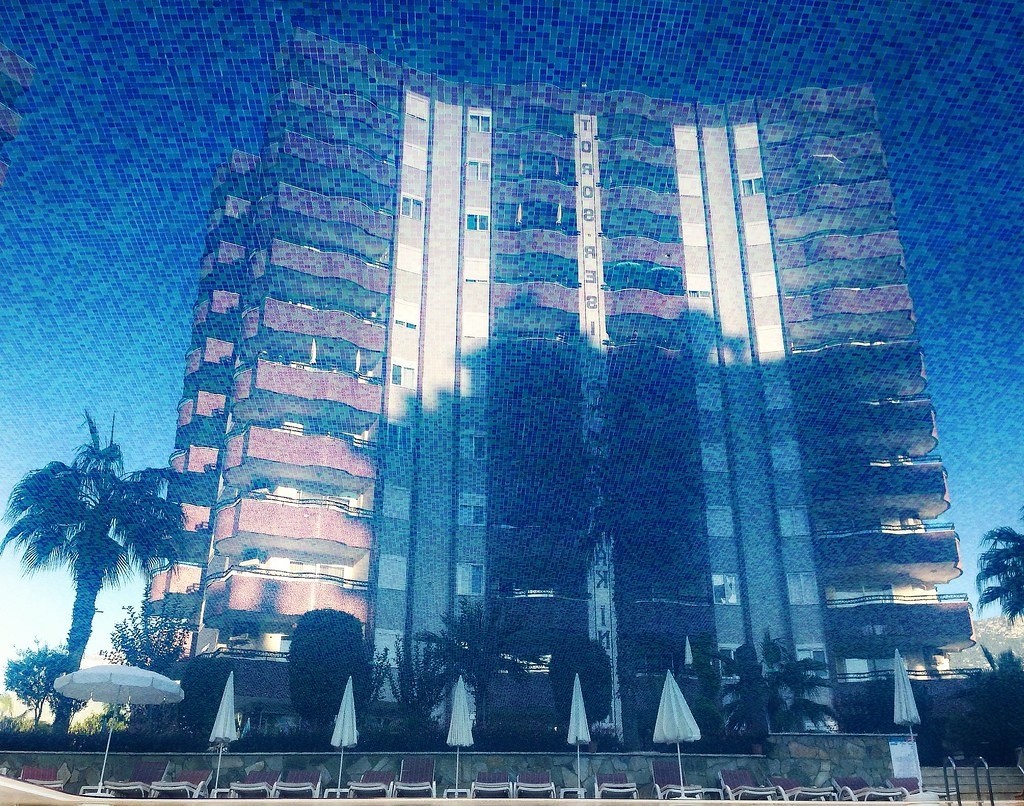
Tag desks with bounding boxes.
[702,788,724,800]
[443,788,470,798]
[79,785,108,798]
[906,792,940,801]
[559,788,587,799]
[324,788,350,799]
[210,788,238,799]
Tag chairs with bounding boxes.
[393,757,436,799]
[830,777,902,801]
[229,771,281,799]
[514,771,556,799]
[594,773,640,799]
[270,771,322,799]
[18,766,63,792]
[470,772,513,799]
[348,770,398,798]
[104,761,171,799]
[649,759,703,799]
[766,777,839,801]
[718,769,777,801]
[885,776,956,801]
[149,768,213,799]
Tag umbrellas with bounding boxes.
[893,648,924,794]
[446,675,474,798]
[653,669,702,797]
[209,670,239,790]
[331,676,358,798]
[568,673,591,798]
[53,665,185,793]
